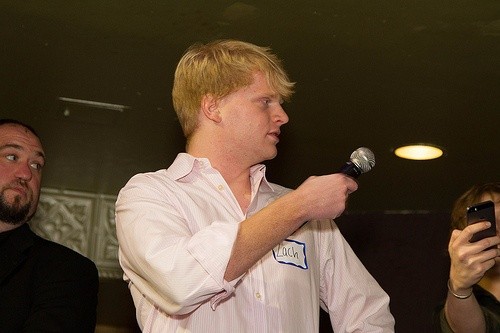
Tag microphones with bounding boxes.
[290,147,375,239]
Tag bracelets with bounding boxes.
[446,278,474,300]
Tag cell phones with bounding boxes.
[467,201,496,257]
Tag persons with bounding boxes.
[114,39,395,332]
[440,179,500,333]
[0,118,100,333]
